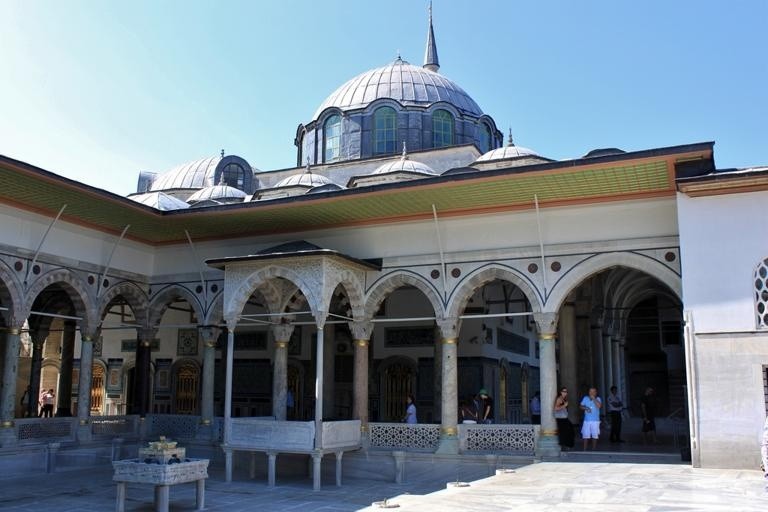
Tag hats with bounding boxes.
[479,389,488,395]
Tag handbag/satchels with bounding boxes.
[553,404,569,419]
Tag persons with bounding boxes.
[461,395,478,424]
[287,385,295,420]
[640,385,664,449]
[606,385,627,443]
[530,390,540,423]
[478,388,496,424]
[400,393,418,423]
[554,386,574,451]
[20,384,31,418]
[43,388,54,417]
[578,386,603,450]
[38,389,47,417]
[473,393,481,410]
